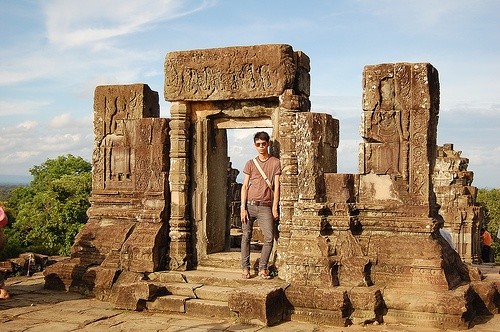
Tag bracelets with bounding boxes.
[240,203,246,208]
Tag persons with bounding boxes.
[479,227,493,264]
[437,213,457,252]
[0,207,12,300]
[239,130,282,281]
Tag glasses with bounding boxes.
[255,141,268,147]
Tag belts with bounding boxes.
[246,200,271,207]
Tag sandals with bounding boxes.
[242,271,250,280]
[260,273,271,280]
